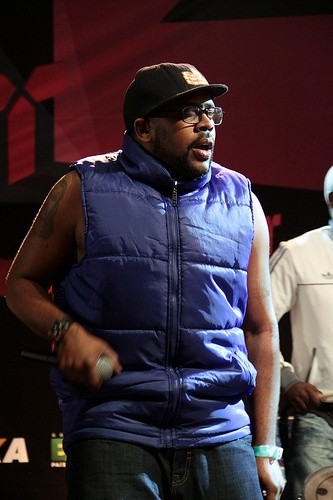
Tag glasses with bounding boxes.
[156,105,224,125]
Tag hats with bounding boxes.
[122,63,228,128]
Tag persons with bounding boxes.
[4,62,287,500]
[270,165,333,500]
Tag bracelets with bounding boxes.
[36,314,73,359]
[251,445,284,460]
